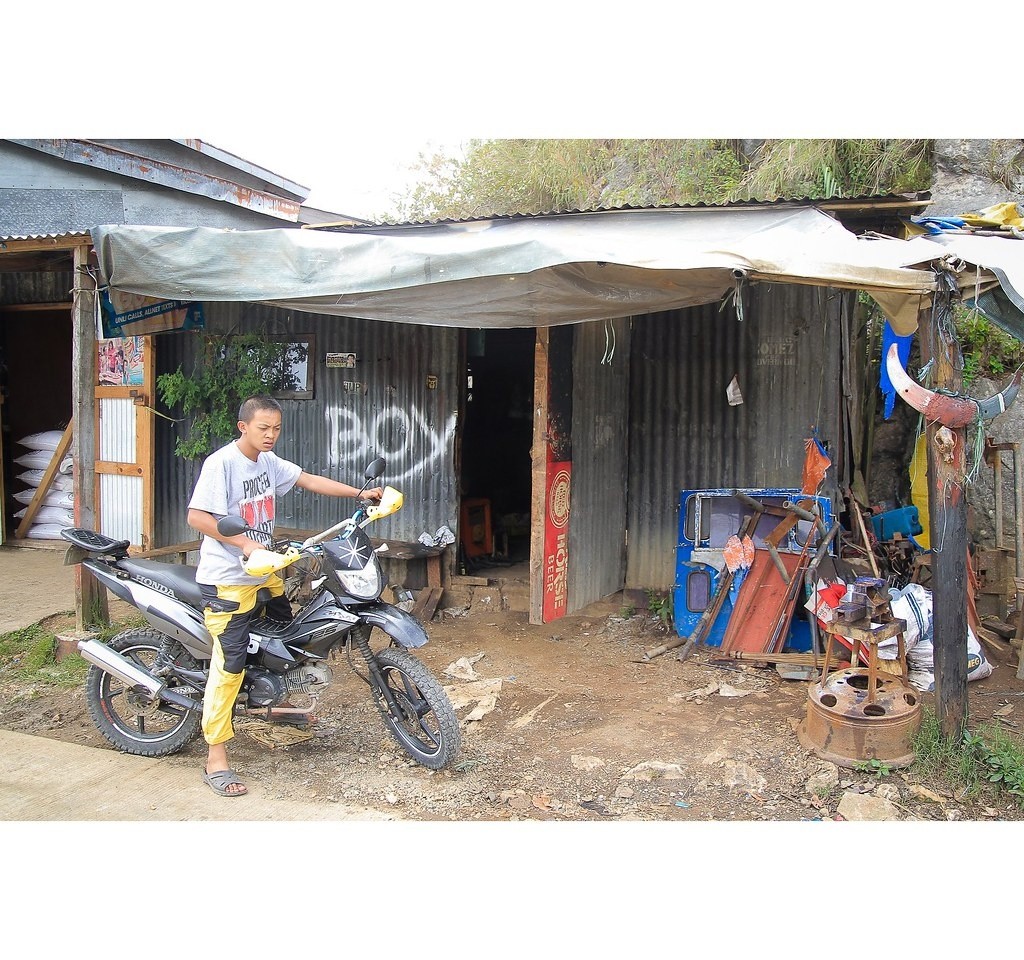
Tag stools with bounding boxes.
[460,498,493,558]
[820,617,907,704]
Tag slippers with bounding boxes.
[256,704,319,725]
[202,769,247,797]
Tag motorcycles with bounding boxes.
[60,457,461,769]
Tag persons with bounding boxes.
[188,394,382,795]
[99,342,124,374]
